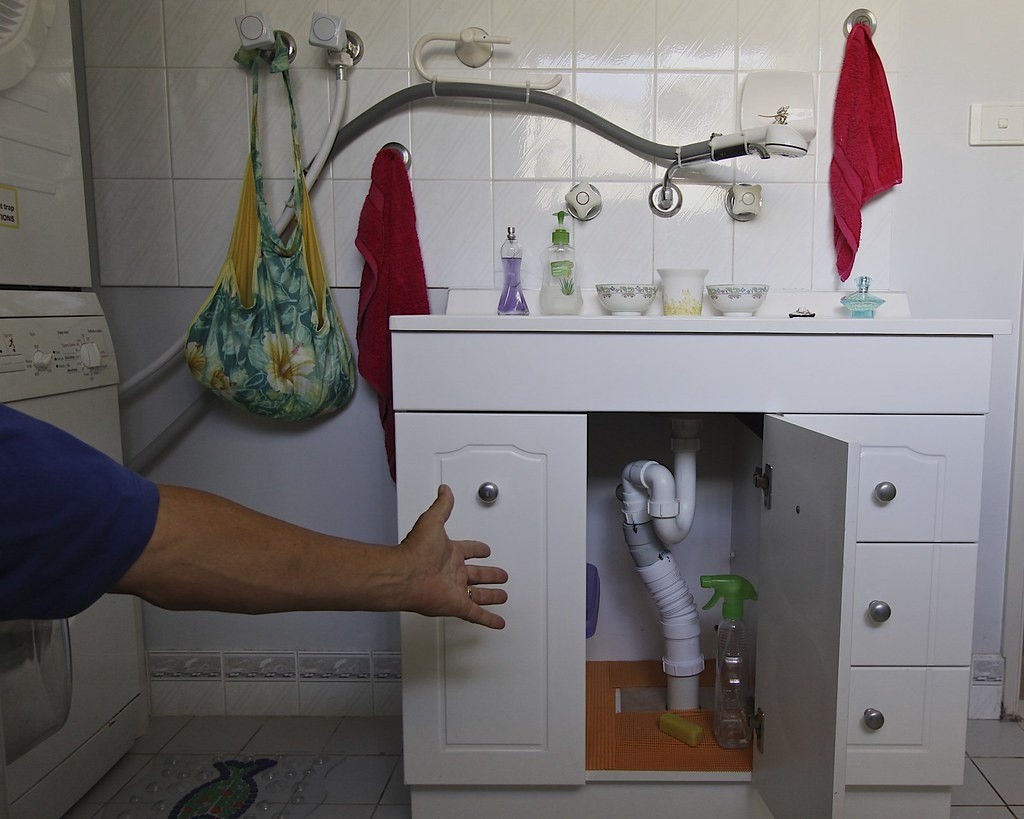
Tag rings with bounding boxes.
[467,587,472,598]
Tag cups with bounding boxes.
[658,269,709,315]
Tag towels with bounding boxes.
[827,21,904,287]
[355,143,434,483]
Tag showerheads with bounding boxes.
[709,124,810,159]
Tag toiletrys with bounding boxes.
[538,210,584,315]
[495,226,531,316]
[840,275,886,320]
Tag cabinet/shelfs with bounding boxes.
[389,325,993,819]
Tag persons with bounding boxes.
[0,384,508,629]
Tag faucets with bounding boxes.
[664,143,771,189]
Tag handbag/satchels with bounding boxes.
[186,32,358,423]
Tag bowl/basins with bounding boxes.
[706,284,769,318]
[596,283,659,316]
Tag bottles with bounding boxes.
[498,226,529,314]
